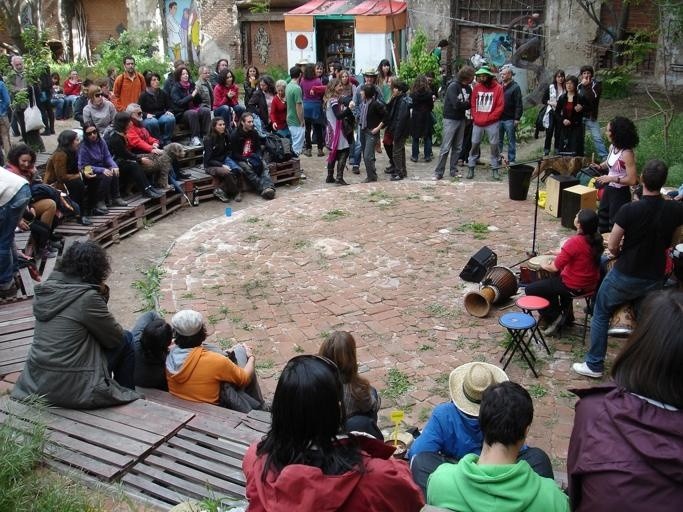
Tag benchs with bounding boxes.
[2,379,272,512]
[2,228,90,375]
[27,180,216,249]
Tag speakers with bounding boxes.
[460,246,496,283]
[544,176,580,218]
[562,184,596,230]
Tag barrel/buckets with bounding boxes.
[508,164,535,200]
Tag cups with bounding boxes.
[84,165,93,178]
[225,207,232,217]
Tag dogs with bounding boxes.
[530,155,593,183]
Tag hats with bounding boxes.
[362,67,378,76]
[449,361,510,417]
[475,66,496,77]
[171,309,203,336]
[58,130,76,145]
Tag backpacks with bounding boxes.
[266,132,292,162]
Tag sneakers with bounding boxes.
[39,234,62,258]
[255,400,272,412]
[158,179,175,191]
[0,273,22,299]
[213,188,242,202]
[304,149,324,157]
[433,157,515,179]
[543,311,566,336]
[573,362,603,378]
[190,136,201,146]
[260,189,274,199]
[76,198,127,225]
[411,150,433,162]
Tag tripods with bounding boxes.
[491,159,561,270]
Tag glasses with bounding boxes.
[133,112,142,116]
[85,130,98,136]
[95,93,104,97]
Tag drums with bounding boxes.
[605,256,637,338]
[601,232,624,251]
[463,265,519,318]
[528,254,560,325]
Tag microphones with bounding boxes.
[557,151,576,155]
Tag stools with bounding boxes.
[515,296,550,354]
[498,312,539,378]
[143,111,215,166]
[35,152,54,181]
[180,157,300,205]
[558,291,596,345]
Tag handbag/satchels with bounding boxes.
[39,91,49,102]
[304,99,322,119]
[23,105,43,133]
[537,105,553,132]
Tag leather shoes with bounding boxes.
[176,173,191,178]
[144,185,166,199]
[40,127,55,135]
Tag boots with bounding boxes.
[326,138,407,184]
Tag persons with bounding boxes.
[567,290,683,510]
[165,2,182,60]
[12,240,146,411]
[428,39,449,78]
[525,65,683,378]
[242,331,425,511]
[408,362,570,510]
[131,309,269,413]
[180,8,190,49]
[299,58,524,185]
[1,55,306,297]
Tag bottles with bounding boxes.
[192,184,200,208]
